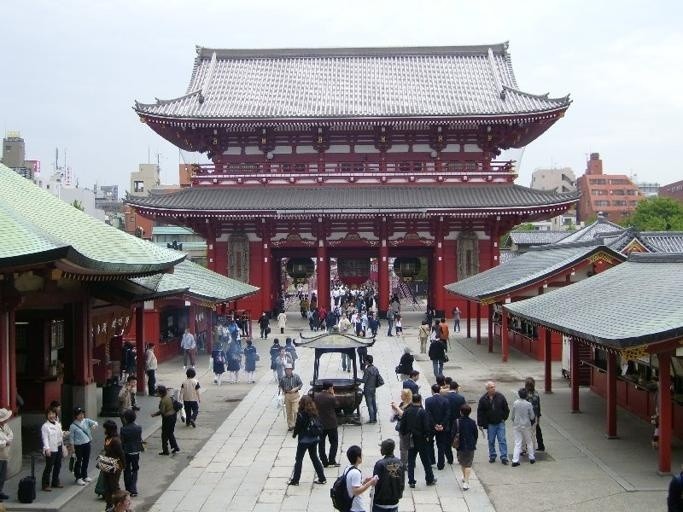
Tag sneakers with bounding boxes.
[43,476,92,491]
[409,451,546,490]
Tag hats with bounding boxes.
[0,408,13,423]
[377,438,396,450]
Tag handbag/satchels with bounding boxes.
[452,432,460,451]
[444,354,449,363]
[170,397,183,412]
[95,454,121,476]
[402,433,415,449]
[61,444,68,458]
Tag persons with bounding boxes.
[40,409,69,493]
[477,377,545,467]
[96,422,123,499]
[104,490,135,512]
[68,407,98,485]
[258,312,270,338]
[241,312,248,335]
[277,310,287,333]
[353,355,379,424]
[277,363,341,486]
[270,338,298,384]
[49,401,70,439]
[120,342,201,456]
[180,328,198,368]
[400,306,462,379]
[666,467,682,512]
[212,321,256,386]
[300,284,404,372]
[326,447,380,512]
[0,408,14,504]
[651,374,675,449]
[368,439,408,512]
[391,370,478,491]
[118,410,141,497]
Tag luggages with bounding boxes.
[95,470,105,497]
[17,456,36,504]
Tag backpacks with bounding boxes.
[375,372,384,388]
[306,417,324,436]
[329,466,362,512]
